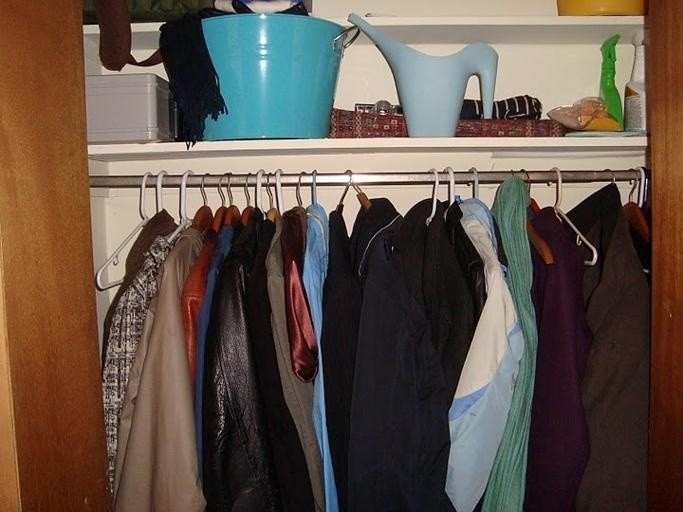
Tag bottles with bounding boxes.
[624,28,646,132]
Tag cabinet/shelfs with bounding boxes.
[82,16,645,153]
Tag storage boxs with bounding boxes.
[86,73,175,143]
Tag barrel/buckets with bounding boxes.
[199,13,359,140]
